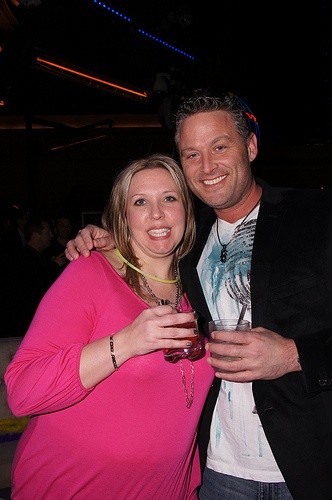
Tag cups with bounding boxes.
[208,318,251,373]
[160,311,203,363]
